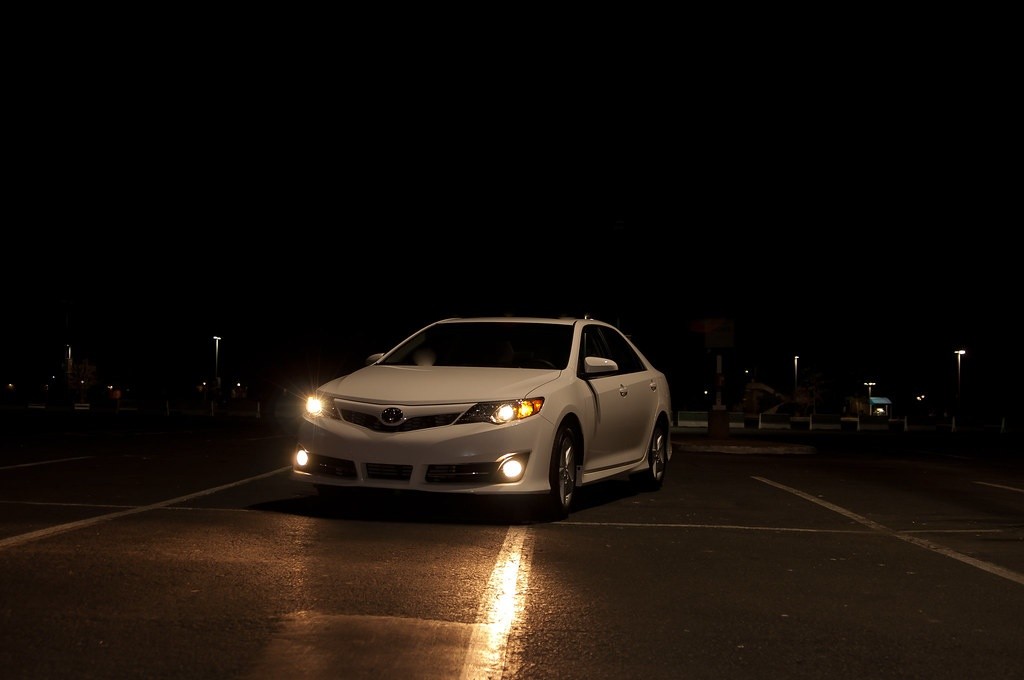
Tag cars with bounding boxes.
[284,316,673,522]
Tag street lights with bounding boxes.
[794,354,800,390]
[954,350,966,388]
[211,336,222,377]
[863,382,876,416]
